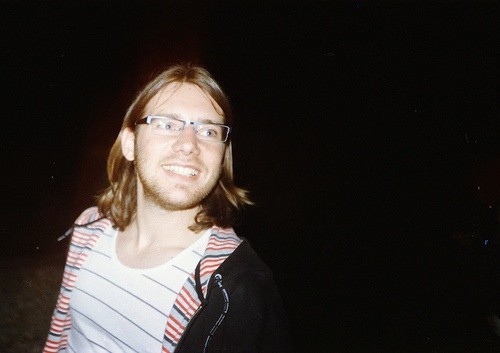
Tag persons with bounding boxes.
[3,58,265,351]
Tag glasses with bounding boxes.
[133,114,231,144]
